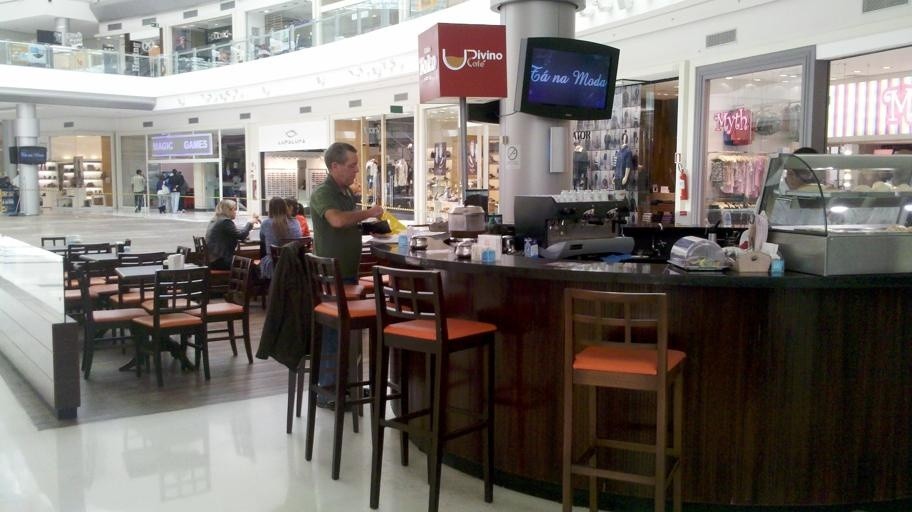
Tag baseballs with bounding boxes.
[677,163,687,200]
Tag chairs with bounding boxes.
[132,268,211,387]
[183,255,255,366]
[42,236,274,335]
[80,275,150,380]
[303,251,413,480]
[370,265,497,512]
[318,387,369,411]
[562,286,687,512]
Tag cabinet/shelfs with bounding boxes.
[523,237,532,258]
[39,156,104,205]
[760,154,912,275]
[426,135,499,214]
[531,240,539,258]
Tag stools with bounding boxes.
[272,245,392,432]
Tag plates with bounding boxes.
[39,156,104,205]
[426,135,499,214]
[760,154,912,275]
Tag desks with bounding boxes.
[115,262,208,379]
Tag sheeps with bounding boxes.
[651,224,667,256]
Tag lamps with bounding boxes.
[370,265,497,512]
[303,251,413,480]
[562,286,687,512]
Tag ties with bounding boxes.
[519,36,620,119]
[8,146,17,164]
[37,29,62,45]
[18,147,47,164]
[466,99,500,126]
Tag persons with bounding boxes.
[611,144,632,190]
[203,196,310,281]
[156,169,188,214]
[131,169,146,213]
[573,144,588,191]
[309,143,384,412]
[754,147,824,225]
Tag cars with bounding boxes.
[560,188,608,202]
[470,242,483,261]
[454,242,471,258]
[481,243,496,261]
[398,233,407,247]
[409,235,428,250]
